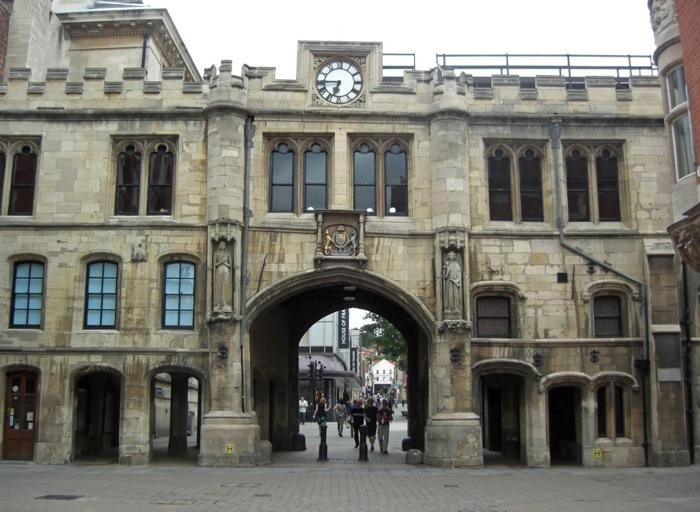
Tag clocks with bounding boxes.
[312,56,365,106]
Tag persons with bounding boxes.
[298,388,394,455]
[213,239,233,308]
[442,250,463,313]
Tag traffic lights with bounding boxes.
[306,361,313,384]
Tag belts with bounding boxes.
[379,422,388,425]
